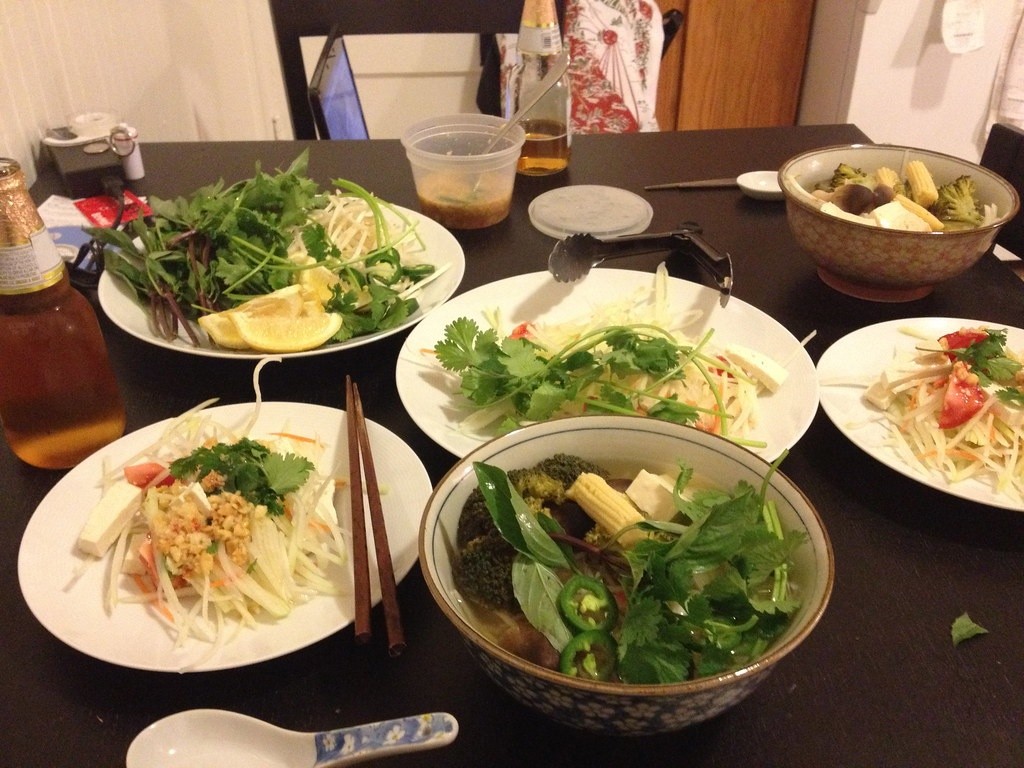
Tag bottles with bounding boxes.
[510,0,572,177]
[0,159,125,470]
[113,125,146,183]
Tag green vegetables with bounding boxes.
[428,307,736,444]
[957,327,1024,408]
[171,439,314,515]
[78,140,434,340]
[469,451,812,684]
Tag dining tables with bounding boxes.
[0,124,1024,768]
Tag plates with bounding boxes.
[393,264,821,467]
[94,200,467,361]
[16,399,433,674]
[820,316,1023,520]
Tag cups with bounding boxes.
[402,113,526,228]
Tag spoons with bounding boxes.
[736,169,784,200]
[123,709,460,768]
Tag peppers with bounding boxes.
[557,577,618,687]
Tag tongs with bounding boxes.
[545,225,735,311]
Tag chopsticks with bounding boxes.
[341,370,406,662]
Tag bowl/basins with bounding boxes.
[773,141,1019,304]
[420,416,835,734]
